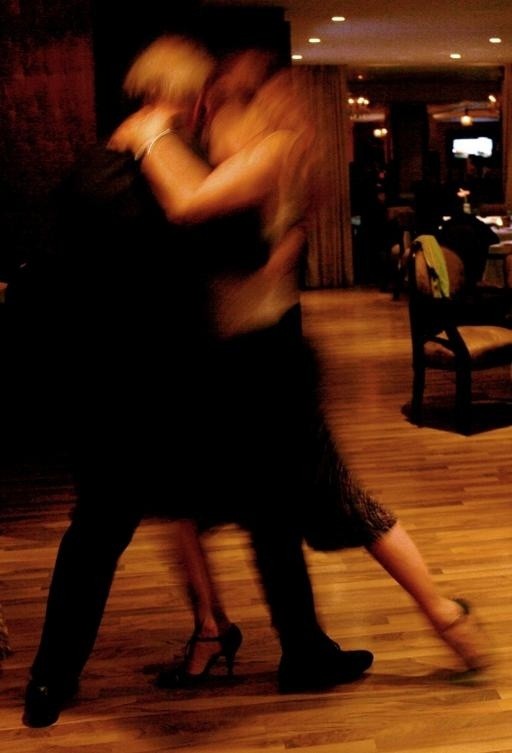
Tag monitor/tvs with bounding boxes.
[452,136,494,160]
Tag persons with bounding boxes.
[23,32,512,729]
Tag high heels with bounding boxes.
[438,598,495,675]
[151,620,243,687]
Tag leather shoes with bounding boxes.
[22,679,80,727]
[278,649,372,695]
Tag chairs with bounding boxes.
[351,203,512,436]
